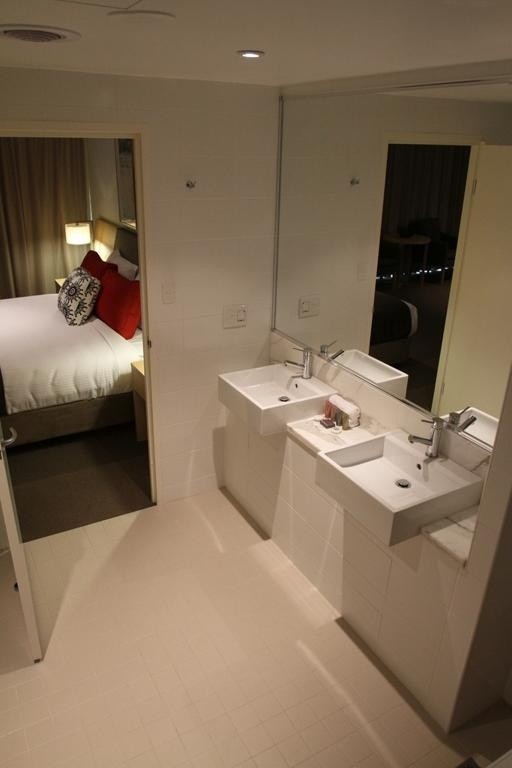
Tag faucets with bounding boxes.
[320,339,345,363]
[411,418,442,458]
[447,407,477,434]
[285,348,313,378]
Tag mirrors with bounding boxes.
[270,77,512,452]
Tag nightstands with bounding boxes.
[132,362,147,441]
[54,278,66,293]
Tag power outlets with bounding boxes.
[299,294,319,318]
[222,304,247,329]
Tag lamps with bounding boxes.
[66,223,92,246]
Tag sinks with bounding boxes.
[317,429,482,514]
[217,363,336,435]
[438,407,499,451]
[326,349,409,397]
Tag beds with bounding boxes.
[0,218,145,449]
[369,290,419,368]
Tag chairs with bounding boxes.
[410,218,457,288]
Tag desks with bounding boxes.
[383,230,431,287]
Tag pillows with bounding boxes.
[58,268,101,326]
[94,269,140,340]
[80,251,118,281]
[108,249,138,281]
